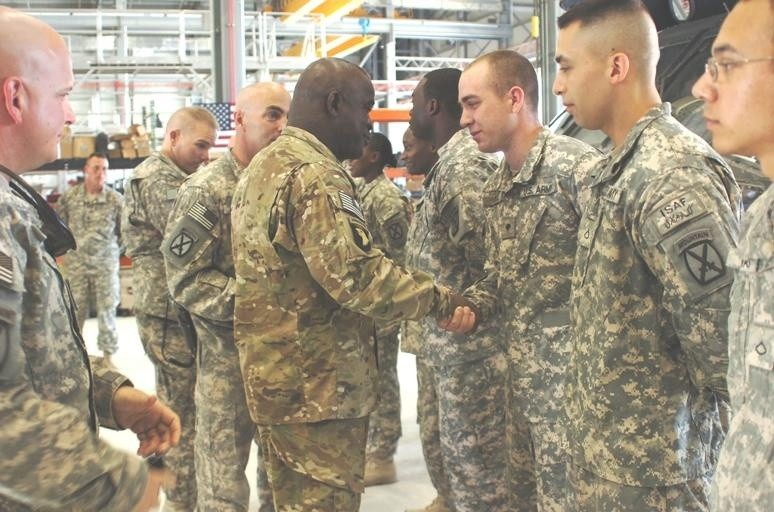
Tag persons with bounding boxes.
[433,48,606,510]
[0,0,182,511]
[346,132,416,488]
[397,122,440,512]
[229,55,482,512]
[53,150,128,367]
[685,0,772,511]
[407,64,507,511]
[554,0,746,511]
[161,78,292,512]
[117,100,218,512]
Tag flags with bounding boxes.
[190,102,236,154]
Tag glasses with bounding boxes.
[705,57,773,83]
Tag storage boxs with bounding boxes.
[60,125,150,160]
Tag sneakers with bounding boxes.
[404,496,448,512]
[362,459,397,486]
[103,352,118,370]
